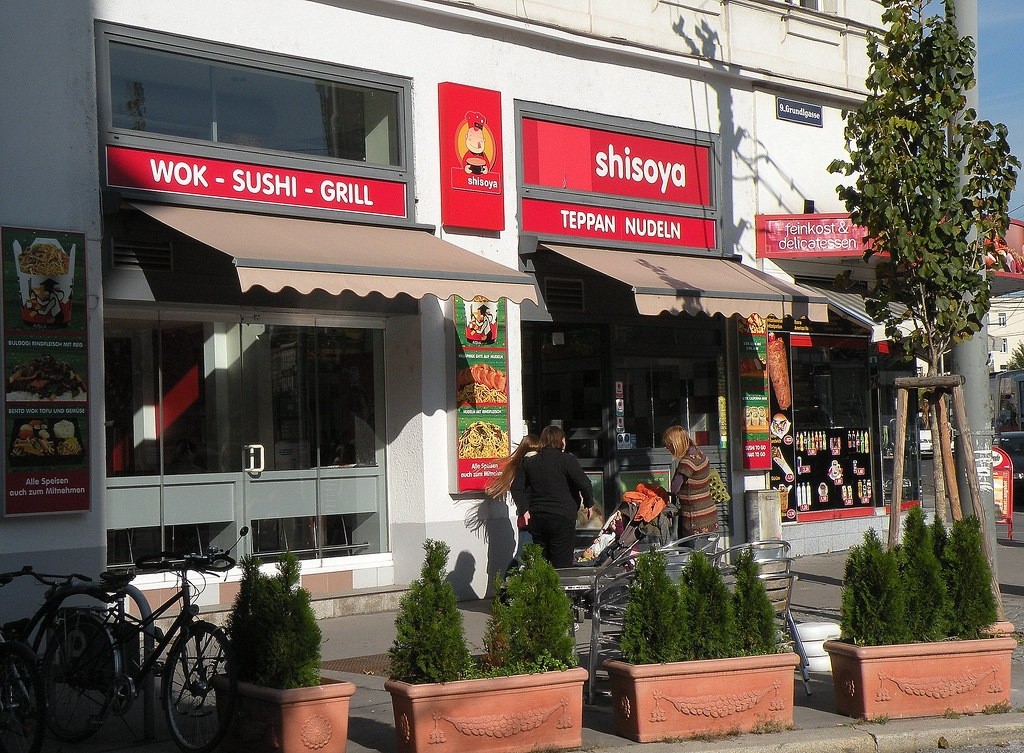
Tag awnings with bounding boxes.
[544,240,832,321]
[124,203,541,306]
[801,275,952,344]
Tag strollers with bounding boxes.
[571,482,679,623]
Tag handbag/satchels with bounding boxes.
[707,469,731,502]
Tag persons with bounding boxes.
[582,510,624,560]
[482,426,595,568]
[174,439,206,474]
[662,425,721,553]
[328,433,355,464]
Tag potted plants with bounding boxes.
[823,504,1016,721]
[384,538,588,753]
[602,544,799,742]
[211,552,356,753]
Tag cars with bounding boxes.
[918,412,957,458]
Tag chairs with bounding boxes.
[106,511,371,577]
[587,532,812,703]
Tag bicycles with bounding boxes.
[40,525,251,753]
[0,565,94,753]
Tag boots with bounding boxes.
[495,559,520,608]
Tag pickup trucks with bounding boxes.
[992,368,1024,513]
